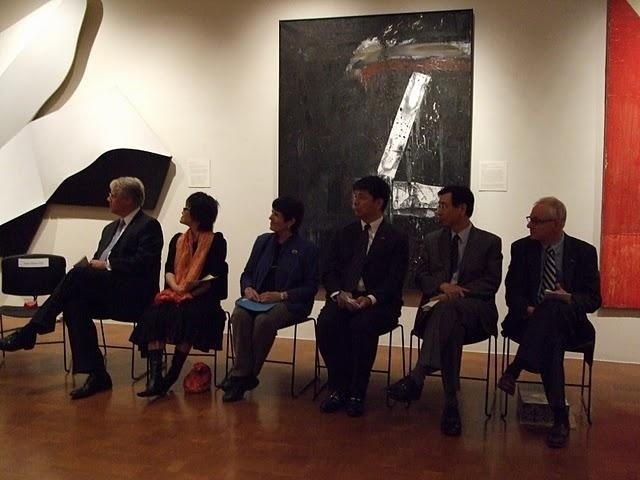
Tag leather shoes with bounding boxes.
[346,396,365,417]
[221,374,250,401]
[440,406,462,437]
[382,375,423,402]
[218,375,260,392]
[69,369,113,399]
[0,326,36,352]
[496,371,516,396]
[547,415,570,449]
[320,391,344,413]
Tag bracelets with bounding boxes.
[282,291,288,300]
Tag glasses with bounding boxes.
[437,203,461,209]
[526,216,554,226]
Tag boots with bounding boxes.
[150,347,186,397]
[136,348,163,403]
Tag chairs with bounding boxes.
[402,331,498,417]
[312,324,405,406]
[502,336,595,426]
[225,318,321,398]
[0,254,71,372]
[146,310,231,389]
[63,316,155,380]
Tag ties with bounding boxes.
[539,247,558,303]
[98,219,126,262]
[448,234,461,284]
[352,223,372,289]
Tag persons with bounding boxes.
[382,185,503,436]
[0,176,164,399]
[127,192,229,402]
[220,195,319,403]
[497,196,602,448]
[314,175,410,418]
